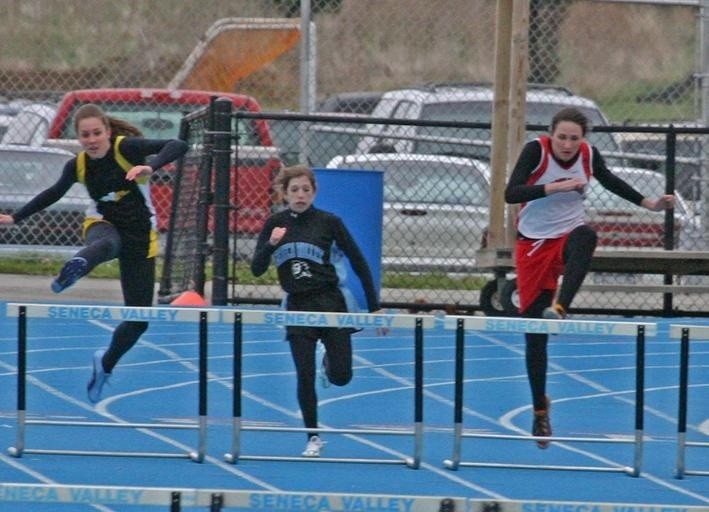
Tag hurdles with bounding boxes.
[0,302,709,510]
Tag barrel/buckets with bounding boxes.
[308,166,382,309]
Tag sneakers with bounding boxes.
[532,396,552,449]
[319,349,331,389]
[50,257,87,292]
[86,349,111,404]
[543,304,566,336]
[301,435,324,458]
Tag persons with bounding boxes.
[0,103,189,404]
[504,107,677,449]
[251,165,389,457]
